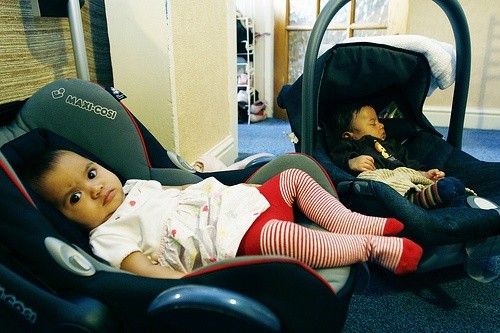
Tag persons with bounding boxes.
[32,149,423,279]
[324,99,466,210]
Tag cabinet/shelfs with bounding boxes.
[236,16,256,125]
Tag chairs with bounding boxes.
[277,0,500,294]
[0,76,359,333]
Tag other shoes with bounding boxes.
[238,90,248,104]
[251,100,265,114]
[239,74,250,85]
[249,111,266,123]
